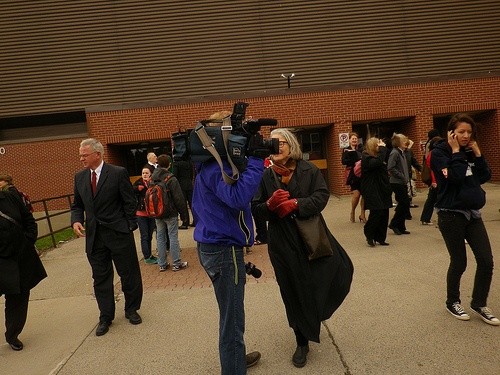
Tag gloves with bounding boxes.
[267,189,290,210]
[272,198,298,217]
[249,133,269,158]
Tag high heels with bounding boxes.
[359,216,367,223]
[350,212,355,222]
[364,232,389,246]
[420,219,433,225]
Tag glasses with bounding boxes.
[278,140,289,148]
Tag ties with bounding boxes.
[92,171,97,196]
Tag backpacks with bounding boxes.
[421,156,432,185]
[15,187,34,213]
[143,175,174,218]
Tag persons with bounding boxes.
[361,137,392,246]
[194,111,271,375]
[0,190,48,350]
[0,173,19,194]
[244,156,271,255]
[251,128,354,366]
[71,138,143,336]
[174,160,196,230]
[421,130,440,225]
[151,155,189,271]
[388,133,422,236]
[342,133,368,224]
[132,153,157,264]
[430,113,500,325]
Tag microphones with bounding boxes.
[245,262,261,278]
[257,117,278,126]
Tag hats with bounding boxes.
[430,136,446,145]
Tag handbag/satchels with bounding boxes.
[296,216,333,261]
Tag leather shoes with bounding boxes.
[125,311,142,324]
[96,321,112,336]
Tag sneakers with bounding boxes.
[172,262,188,271]
[470,306,500,325]
[159,263,170,271]
[446,300,470,320]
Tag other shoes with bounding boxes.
[144,255,159,264]
[178,224,188,229]
[254,239,265,245]
[388,225,410,234]
[247,351,261,367]
[6,337,24,350]
[292,345,310,368]
[189,223,195,227]
[410,203,419,208]
